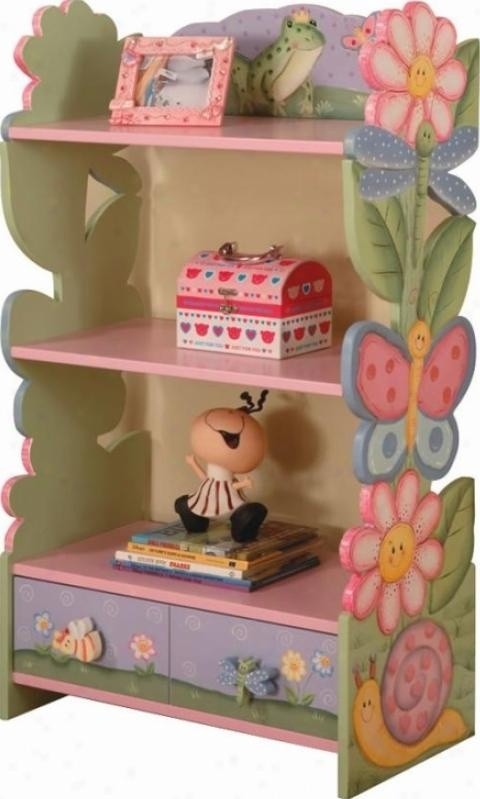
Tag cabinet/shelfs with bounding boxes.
[0,106,480,796]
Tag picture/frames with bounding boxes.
[108,34,236,126]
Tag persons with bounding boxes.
[174,385,270,544]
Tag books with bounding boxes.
[110,517,327,595]
